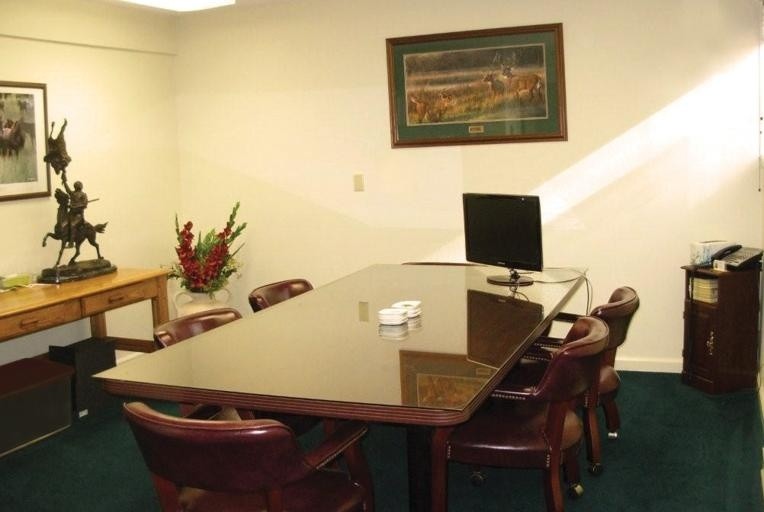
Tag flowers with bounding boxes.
[161,202,246,293]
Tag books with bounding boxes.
[688,277,717,305]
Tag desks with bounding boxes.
[94,261,591,512]
[0,265,171,362]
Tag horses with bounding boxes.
[0,119,24,160]
[41,188,107,266]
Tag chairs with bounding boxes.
[464,315,610,511]
[543,287,639,438]
[153,307,361,470]
[122,399,373,512]
[248,274,314,313]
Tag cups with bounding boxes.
[378,300,422,325]
[378,317,421,340]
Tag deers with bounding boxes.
[501,63,542,103]
[481,72,504,96]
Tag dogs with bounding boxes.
[409,88,453,125]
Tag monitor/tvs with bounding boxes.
[463,193,543,284]
[466,284,544,370]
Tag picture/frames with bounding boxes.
[0,79,51,202]
[385,23,567,147]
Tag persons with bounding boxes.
[63,179,87,248]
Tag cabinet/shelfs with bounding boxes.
[681,261,760,392]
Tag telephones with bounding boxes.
[710,244,764,271]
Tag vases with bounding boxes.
[168,288,233,315]
